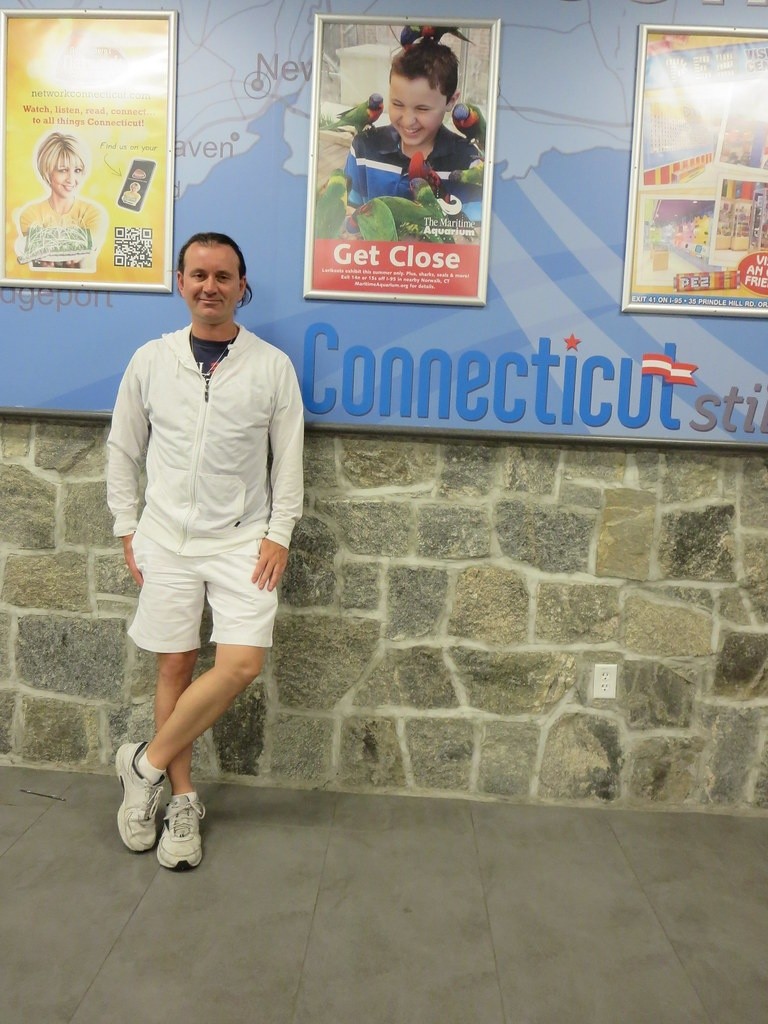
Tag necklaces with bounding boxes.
[190,324,237,378]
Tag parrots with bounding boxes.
[315,25,487,244]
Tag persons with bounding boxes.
[122,182,141,206]
[19,133,110,274]
[106,232,304,872]
[343,37,483,243]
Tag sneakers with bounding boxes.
[158,790,206,872]
[115,742,166,854]
